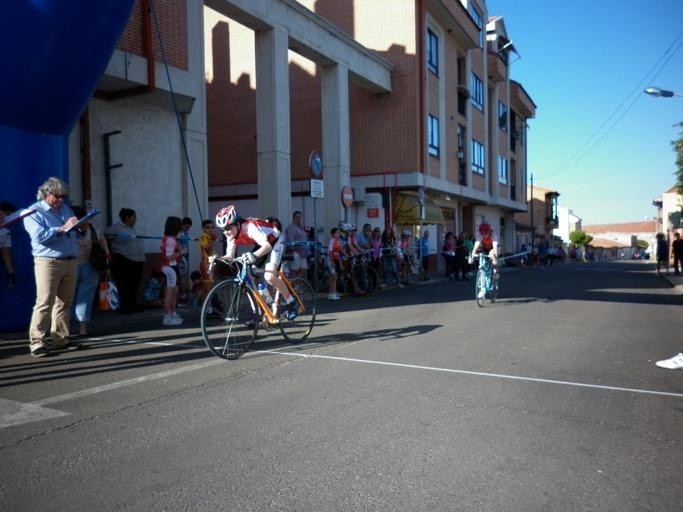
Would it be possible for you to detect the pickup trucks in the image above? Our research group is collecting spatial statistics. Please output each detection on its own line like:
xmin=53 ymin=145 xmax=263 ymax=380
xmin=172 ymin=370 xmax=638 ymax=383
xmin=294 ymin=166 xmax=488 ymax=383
xmin=68 ymin=205 xmax=167 ymax=309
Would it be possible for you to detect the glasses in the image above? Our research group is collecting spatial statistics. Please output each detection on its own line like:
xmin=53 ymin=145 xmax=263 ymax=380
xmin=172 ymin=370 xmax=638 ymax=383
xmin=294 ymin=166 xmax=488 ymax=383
xmin=220 ymin=227 xmax=230 ymax=232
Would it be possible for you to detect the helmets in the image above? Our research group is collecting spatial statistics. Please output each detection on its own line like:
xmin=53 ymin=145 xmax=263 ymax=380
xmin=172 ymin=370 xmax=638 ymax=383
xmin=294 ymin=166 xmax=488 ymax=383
xmin=215 ymin=204 xmax=239 ymax=228
xmin=340 ymin=223 xmax=353 ymax=232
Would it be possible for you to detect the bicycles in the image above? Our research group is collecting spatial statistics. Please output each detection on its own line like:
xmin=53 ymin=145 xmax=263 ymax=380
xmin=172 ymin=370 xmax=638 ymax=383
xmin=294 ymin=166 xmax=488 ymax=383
xmin=200 ymin=254 xmax=317 ymax=364
xmin=467 ymin=250 xmax=500 ymax=308
xmin=309 ymin=251 xmax=424 ymax=296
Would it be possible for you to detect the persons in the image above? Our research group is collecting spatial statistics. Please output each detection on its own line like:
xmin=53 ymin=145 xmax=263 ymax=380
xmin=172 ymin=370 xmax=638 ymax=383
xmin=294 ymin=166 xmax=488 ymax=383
xmin=22 ymin=176 xmax=89 ymax=358
xmin=672 ymin=232 xmax=683 ymax=276
xmin=655 ymin=233 xmax=670 ymax=277
xmin=0 ymin=200 xmax=18 ymax=290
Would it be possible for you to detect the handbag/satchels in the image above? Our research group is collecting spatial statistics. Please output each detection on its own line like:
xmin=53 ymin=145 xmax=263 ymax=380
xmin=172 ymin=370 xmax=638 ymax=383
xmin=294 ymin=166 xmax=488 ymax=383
xmin=89 ymin=242 xmax=108 ymax=273
xmin=99 ymin=281 xmax=121 ymax=310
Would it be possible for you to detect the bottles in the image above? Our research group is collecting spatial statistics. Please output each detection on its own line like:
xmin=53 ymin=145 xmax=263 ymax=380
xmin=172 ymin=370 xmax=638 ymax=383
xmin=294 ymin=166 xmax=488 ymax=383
xmin=256 ymin=282 xmax=273 ymax=306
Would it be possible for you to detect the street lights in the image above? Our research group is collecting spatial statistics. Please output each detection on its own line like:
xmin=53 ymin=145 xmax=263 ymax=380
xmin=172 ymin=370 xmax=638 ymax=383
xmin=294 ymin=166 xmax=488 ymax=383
xmin=645 ymin=87 xmax=682 ymax=102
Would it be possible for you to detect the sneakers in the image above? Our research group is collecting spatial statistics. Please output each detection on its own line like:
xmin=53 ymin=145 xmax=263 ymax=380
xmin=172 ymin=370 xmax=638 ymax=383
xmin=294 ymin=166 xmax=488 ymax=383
xmin=163 ymin=313 xmax=184 ymax=325
xmin=286 ymin=300 xmax=298 ymax=321
xmin=328 ymin=295 xmax=340 ymax=300
xmin=65 ymin=342 xmax=79 ymax=350
xmin=354 ymin=289 xmax=367 ymax=297
xmin=32 ymin=347 xmax=51 ymax=358
xmin=245 ymin=313 xmax=264 ymax=327
xmin=655 ymin=352 xmax=683 ymax=370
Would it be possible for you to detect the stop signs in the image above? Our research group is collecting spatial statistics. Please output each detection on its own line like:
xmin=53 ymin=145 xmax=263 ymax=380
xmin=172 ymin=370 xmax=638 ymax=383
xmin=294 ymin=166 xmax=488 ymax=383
xmin=342 ymin=185 xmax=353 ymax=208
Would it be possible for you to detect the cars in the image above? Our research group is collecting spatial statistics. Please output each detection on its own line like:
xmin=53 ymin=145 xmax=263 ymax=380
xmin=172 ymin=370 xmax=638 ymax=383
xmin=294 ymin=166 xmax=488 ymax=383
xmin=632 ymin=250 xmax=648 ymax=259
xmin=546 ymin=248 xmax=566 ymax=261
